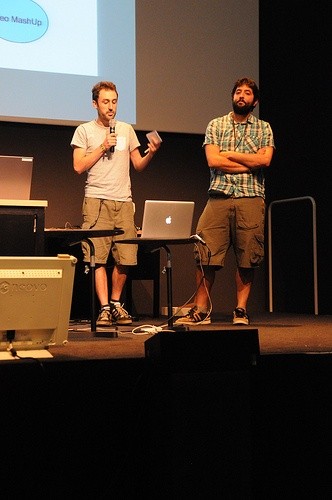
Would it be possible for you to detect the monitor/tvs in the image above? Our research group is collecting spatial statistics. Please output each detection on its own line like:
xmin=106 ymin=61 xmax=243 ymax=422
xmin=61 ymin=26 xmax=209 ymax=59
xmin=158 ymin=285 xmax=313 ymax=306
xmin=0 ymin=254 xmax=77 ymax=360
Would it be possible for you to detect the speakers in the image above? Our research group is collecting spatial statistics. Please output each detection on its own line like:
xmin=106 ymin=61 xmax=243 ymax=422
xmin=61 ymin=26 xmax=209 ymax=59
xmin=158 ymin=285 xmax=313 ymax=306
xmin=144 ymin=328 xmax=260 ymax=367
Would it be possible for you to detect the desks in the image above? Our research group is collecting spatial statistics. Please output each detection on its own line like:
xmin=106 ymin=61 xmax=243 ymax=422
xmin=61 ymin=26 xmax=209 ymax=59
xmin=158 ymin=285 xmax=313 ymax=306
xmin=45 ymin=229 xmax=126 ymax=338
xmin=0 ymin=198 xmax=48 ymax=257
xmin=112 ymin=236 xmax=199 ymax=330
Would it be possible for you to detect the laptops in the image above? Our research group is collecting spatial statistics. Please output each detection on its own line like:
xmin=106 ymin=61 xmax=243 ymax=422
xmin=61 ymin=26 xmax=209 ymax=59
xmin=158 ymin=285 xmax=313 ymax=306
xmin=140 ymin=200 xmax=194 ymax=239
xmin=0 ymin=156 xmax=33 ymax=200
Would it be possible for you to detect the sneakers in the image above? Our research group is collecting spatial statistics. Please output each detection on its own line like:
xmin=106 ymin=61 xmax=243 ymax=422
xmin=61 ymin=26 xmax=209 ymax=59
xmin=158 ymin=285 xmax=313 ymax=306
xmin=232 ymin=306 xmax=248 ymax=325
xmin=110 ymin=303 xmax=132 ymax=325
xmin=175 ymin=306 xmax=211 ymax=324
xmin=96 ymin=307 xmax=112 ymax=326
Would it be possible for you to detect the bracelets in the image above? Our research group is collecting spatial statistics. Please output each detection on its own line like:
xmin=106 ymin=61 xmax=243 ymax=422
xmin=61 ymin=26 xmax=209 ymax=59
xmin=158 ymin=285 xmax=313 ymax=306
xmin=100 ymin=144 xmax=106 ymax=153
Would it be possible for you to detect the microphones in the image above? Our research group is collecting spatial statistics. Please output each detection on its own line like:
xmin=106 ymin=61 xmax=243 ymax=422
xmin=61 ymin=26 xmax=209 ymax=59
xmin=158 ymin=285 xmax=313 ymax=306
xmin=109 ymin=119 xmax=117 ymax=152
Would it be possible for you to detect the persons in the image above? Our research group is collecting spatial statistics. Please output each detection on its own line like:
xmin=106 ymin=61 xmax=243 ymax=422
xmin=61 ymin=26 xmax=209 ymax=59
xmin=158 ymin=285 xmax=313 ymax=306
xmin=175 ymin=77 xmax=274 ymax=324
xmin=70 ymin=81 xmax=160 ymax=325
